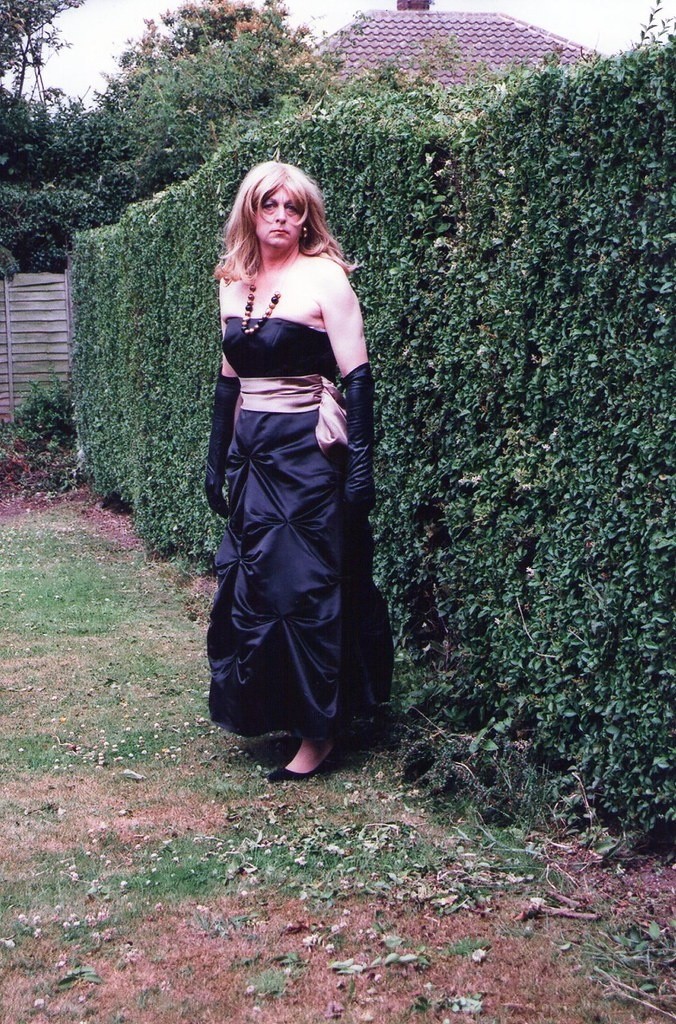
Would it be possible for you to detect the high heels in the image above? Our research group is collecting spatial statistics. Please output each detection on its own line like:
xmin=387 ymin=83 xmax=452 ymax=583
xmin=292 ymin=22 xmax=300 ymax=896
xmin=267 ymin=739 xmax=340 ymax=781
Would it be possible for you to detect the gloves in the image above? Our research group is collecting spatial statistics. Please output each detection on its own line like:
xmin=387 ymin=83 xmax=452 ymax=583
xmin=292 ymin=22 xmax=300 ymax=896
xmin=204 ymin=365 xmax=243 ymax=518
xmin=339 ymin=362 xmax=377 ymax=519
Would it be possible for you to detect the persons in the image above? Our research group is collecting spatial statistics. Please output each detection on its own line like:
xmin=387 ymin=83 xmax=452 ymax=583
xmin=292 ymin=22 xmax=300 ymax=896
xmin=207 ymin=162 xmax=394 ymax=781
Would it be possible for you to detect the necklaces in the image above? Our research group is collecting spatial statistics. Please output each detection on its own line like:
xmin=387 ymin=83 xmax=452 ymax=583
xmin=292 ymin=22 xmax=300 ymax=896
xmin=242 ymin=285 xmax=281 ymax=335
